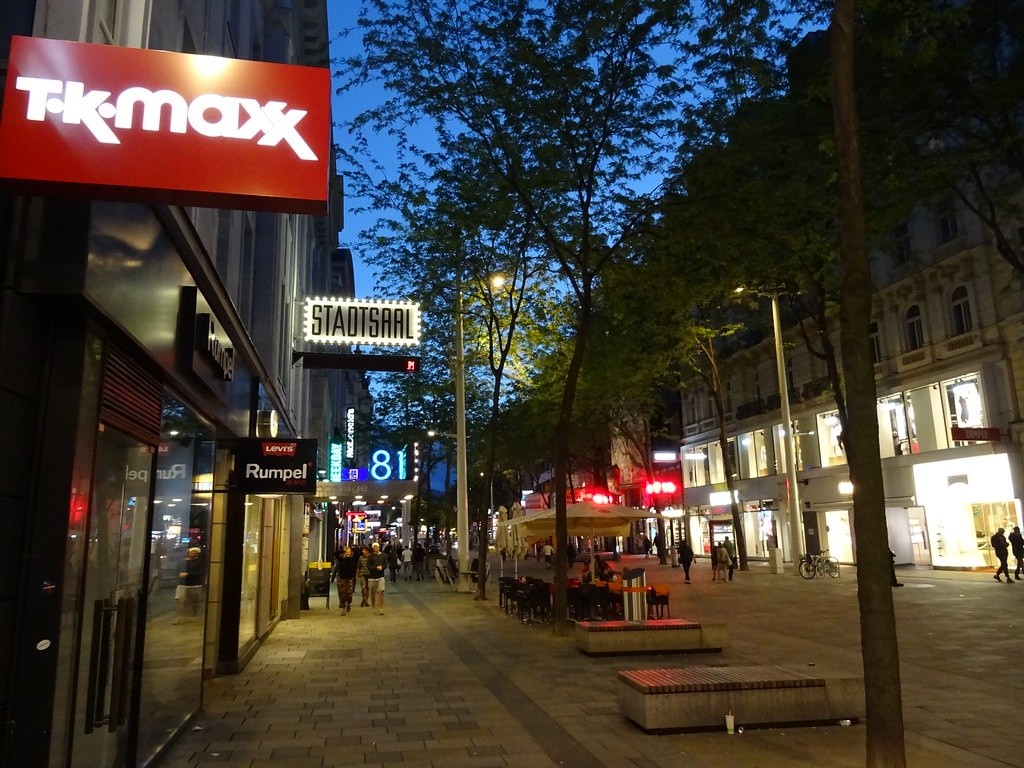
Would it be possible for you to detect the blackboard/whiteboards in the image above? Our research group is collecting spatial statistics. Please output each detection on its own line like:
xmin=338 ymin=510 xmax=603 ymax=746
xmin=307 ymin=567 xmax=330 ymax=596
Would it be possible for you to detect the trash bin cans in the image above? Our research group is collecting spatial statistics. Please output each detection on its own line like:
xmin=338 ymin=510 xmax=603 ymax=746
xmin=671 ymin=546 xmax=680 ymax=567
xmin=622 ymin=567 xmax=647 ymax=621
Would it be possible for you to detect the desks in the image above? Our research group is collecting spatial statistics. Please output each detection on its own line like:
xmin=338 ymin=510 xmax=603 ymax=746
xmin=460 ymin=572 xmax=476 ymax=592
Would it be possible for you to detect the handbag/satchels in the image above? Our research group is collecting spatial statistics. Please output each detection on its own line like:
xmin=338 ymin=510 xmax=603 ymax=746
xmin=726 ymin=553 xmax=732 ymax=567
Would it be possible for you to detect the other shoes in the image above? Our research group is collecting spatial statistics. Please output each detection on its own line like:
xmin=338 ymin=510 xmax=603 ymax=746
xmin=188 ymin=616 xmax=196 ymax=623
xmin=712 ymin=578 xmax=716 ymax=582
xmin=346 ymin=605 xmax=351 ymax=612
xmin=361 ymin=601 xmax=364 ymax=607
xmin=173 ymin=617 xmax=180 ymax=624
xmin=365 ymin=602 xmax=369 ymax=607
xmin=404 ymin=577 xmax=407 ymax=581
xmin=1007 ymin=578 xmax=1014 ymax=583
xmin=1015 ymin=577 xmax=1023 ymax=580
xmin=416 ymin=579 xmax=420 ymax=581
xmin=379 ymin=609 xmax=384 ymax=615
xmin=994 ymin=575 xmax=1002 ymax=582
xmin=341 ymin=611 xmax=346 ymax=616
xmin=421 ymin=574 xmax=424 ymax=580
xmin=685 ymin=581 xmax=691 ymax=584
xmin=409 ymin=575 xmax=412 ymax=580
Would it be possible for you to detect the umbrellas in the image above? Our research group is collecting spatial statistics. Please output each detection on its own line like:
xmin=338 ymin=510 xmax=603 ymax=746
xmin=500 ymin=503 xmax=666 ymax=583
xmin=510 ymin=501 xmax=529 ymax=580
xmin=495 ymin=506 xmax=510 ymax=578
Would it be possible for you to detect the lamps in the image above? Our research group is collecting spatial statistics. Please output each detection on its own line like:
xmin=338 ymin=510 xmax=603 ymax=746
xmin=317 ymin=467 xmax=327 ymax=474
xmin=316 ymin=476 xmax=330 ymax=483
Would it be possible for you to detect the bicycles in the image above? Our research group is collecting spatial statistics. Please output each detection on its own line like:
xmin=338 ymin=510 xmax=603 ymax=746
xmin=799 ymin=549 xmax=838 ymax=579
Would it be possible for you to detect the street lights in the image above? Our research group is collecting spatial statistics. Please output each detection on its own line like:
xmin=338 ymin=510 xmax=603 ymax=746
xmin=392 ymin=506 xmax=400 ymax=541
xmin=480 ymin=473 xmax=493 ymax=547
xmin=428 ymin=270 xmax=506 ymax=594
xmin=733 ymin=286 xmax=805 ymax=580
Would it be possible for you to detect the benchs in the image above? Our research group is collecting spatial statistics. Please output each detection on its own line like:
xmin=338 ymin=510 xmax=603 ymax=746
xmin=618 ymin=663 xmax=870 ymax=735
xmin=574 ymin=616 xmax=727 ymax=651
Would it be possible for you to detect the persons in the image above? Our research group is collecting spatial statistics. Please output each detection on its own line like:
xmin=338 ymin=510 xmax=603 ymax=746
xmin=644 ymin=533 xmax=663 ymax=559
xmin=991 ymin=528 xmax=1015 ymax=584
xmin=761 ymin=446 xmax=768 ymax=471
xmin=172 ymin=547 xmax=204 ymax=624
xmin=334 ymin=538 xmax=438 ymax=615
xmin=710 ymin=536 xmax=736 ymax=583
xmin=502 ymin=541 xmax=554 ymax=570
xmin=566 ymin=537 xmax=598 ymax=568
xmin=890 ymin=549 xmax=904 ymax=586
xmin=1008 ymin=527 xmax=1024 ymax=580
xmin=830 ymin=422 xmax=845 ymax=456
xmin=677 ymin=541 xmax=696 ymax=584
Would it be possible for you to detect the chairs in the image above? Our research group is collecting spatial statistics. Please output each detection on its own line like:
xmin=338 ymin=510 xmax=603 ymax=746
xmin=434 ymin=557 xmax=492 ymax=593
xmin=499 ymin=575 xmax=670 ymax=627
